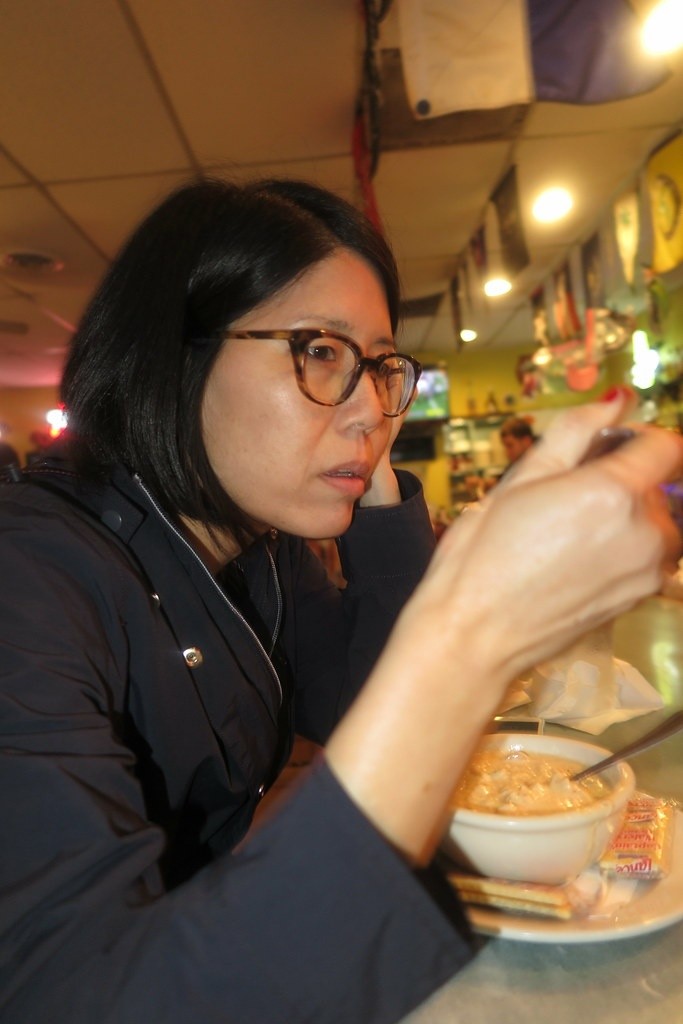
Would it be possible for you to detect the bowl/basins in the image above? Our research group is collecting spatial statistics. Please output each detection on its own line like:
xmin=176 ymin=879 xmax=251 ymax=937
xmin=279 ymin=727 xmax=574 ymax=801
xmin=441 ymin=732 xmax=635 ymax=887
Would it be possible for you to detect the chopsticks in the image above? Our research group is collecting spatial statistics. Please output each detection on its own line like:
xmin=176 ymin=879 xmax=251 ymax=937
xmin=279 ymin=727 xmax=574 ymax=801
xmin=448 ymin=876 xmax=571 ymax=920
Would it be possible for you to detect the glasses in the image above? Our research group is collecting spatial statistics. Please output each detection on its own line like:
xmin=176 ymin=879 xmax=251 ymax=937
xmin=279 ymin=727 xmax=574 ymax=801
xmin=227 ymin=325 xmax=420 ymax=418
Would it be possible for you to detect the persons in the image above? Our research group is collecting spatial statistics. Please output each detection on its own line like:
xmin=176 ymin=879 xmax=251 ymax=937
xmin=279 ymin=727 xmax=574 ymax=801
xmin=0 ymin=171 xmax=683 ymax=1023
xmin=465 ymin=417 xmax=542 ymax=500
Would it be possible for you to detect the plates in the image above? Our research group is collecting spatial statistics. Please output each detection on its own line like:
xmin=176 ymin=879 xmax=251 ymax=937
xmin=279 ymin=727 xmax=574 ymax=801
xmin=421 ymin=788 xmax=683 ymax=944
